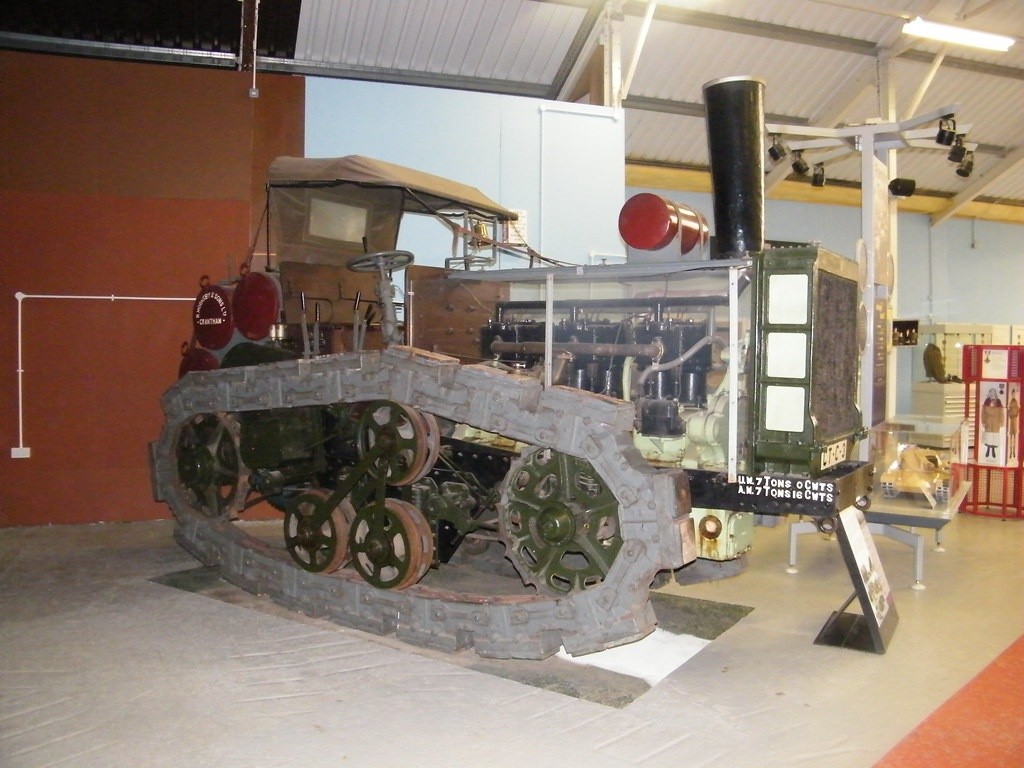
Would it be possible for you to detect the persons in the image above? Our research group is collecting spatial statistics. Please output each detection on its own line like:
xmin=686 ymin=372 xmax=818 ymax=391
xmin=1008 ymin=388 xmax=1018 ymax=458
xmin=982 ymin=389 xmax=1004 ymax=458
xmin=893 ymin=327 xmax=917 ymax=345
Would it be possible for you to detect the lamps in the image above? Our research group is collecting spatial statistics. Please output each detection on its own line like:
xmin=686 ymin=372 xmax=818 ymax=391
xmin=812 ymin=160 xmax=825 ymax=187
xmin=768 ymin=133 xmax=787 ymax=162
xmin=888 ymin=178 xmax=916 ymax=196
xmin=956 ymin=151 xmax=974 ymax=177
xmin=936 ymin=114 xmax=956 ymax=146
xmin=791 ymin=149 xmax=810 ymax=176
xmin=948 ymin=134 xmax=968 ymax=163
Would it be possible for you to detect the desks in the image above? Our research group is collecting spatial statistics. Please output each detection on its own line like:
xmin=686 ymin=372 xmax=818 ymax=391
xmin=786 ymin=480 xmax=973 ymax=590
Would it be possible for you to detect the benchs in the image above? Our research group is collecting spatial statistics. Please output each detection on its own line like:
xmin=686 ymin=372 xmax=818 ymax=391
xmin=280 ymin=263 xmax=405 ymax=354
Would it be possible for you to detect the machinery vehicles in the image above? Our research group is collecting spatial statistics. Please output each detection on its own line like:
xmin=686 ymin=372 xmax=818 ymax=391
xmin=147 ymin=76 xmax=875 ymax=661
